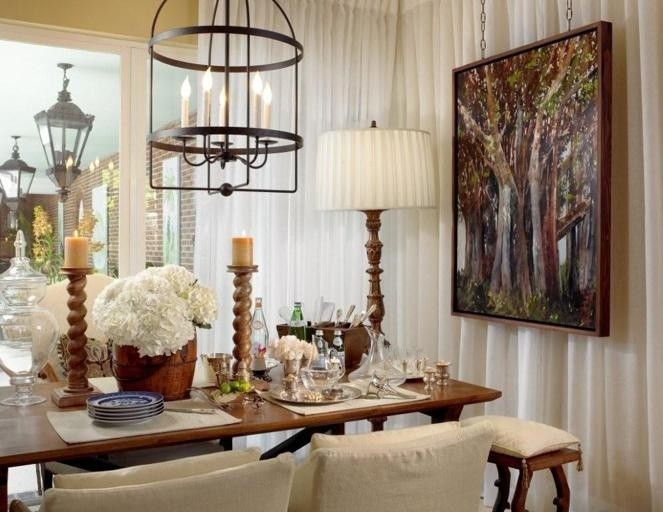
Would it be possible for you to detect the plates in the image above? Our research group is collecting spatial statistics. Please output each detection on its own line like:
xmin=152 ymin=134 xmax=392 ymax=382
xmin=87 ymin=391 xmax=165 ymax=426
xmin=268 ymin=383 xmax=362 ymax=404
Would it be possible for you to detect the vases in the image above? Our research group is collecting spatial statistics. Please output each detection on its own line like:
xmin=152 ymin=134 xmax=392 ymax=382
xmin=113 ymin=337 xmax=198 ymax=402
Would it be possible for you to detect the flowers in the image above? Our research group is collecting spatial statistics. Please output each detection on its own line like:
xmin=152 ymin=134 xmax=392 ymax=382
xmin=92 ymin=264 xmax=218 ymax=361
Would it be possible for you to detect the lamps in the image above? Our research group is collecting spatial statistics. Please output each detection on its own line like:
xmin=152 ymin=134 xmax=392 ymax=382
xmin=316 ymin=120 xmax=440 ymax=345
xmin=34 ymin=62 xmax=94 ymax=206
xmin=0 ymin=135 xmax=35 ymax=232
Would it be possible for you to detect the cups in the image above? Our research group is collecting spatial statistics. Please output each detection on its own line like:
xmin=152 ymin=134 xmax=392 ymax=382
xmin=200 ymin=353 xmax=232 ymax=384
xmin=300 ymin=370 xmax=340 ymax=396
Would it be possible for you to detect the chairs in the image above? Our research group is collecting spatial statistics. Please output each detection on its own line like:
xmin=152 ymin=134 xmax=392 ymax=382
xmin=9 ymin=452 xmax=294 ymax=512
xmin=291 ymin=416 xmax=495 ymax=512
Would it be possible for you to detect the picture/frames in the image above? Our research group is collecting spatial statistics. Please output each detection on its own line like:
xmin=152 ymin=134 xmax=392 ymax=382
xmin=161 ymin=156 xmax=182 ymax=269
xmin=450 ymin=22 xmax=611 ymax=338
xmin=88 ymin=185 xmax=108 ymax=273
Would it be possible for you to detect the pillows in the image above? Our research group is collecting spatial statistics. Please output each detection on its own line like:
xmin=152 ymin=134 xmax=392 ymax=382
xmin=459 ymin=414 xmax=585 ymax=487
xmin=52 ymin=447 xmax=261 ymax=490
xmin=312 ymin=420 xmax=458 ymax=457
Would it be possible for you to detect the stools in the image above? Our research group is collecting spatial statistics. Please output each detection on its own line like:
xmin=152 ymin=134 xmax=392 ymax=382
xmin=461 ymin=447 xmax=582 ymax=512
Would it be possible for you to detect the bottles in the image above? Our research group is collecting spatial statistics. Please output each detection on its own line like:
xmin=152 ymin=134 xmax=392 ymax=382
xmin=236 ymin=359 xmax=249 ymax=384
xmin=347 ymin=326 xmax=406 ymax=392
xmin=289 ymin=301 xmax=307 ymax=343
xmin=314 ymin=330 xmax=328 ymax=357
xmin=251 ymin=297 xmax=269 ymax=357
xmin=330 ymin=330 xmax=345 ymax=371
xmin=307 ymin=334 xmax=346 ymax=383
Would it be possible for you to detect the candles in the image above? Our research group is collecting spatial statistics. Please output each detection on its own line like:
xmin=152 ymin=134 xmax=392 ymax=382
xmin=219 ymin=89 xmax=227 ymax=124
xmin=233 ymin=228 xmax=254 ymax=266
xmin=180 ymin=75 xmax=191 ymax=129
xmin=252 ymin=70 xmax=263 ymax=129
xmin=63 ymin=230 xmax=90 ymax=271
xmin=262 ymin=80 xmax=274 ymax=128
xmin=200 ymin=67 xmax=213 ymax=126
